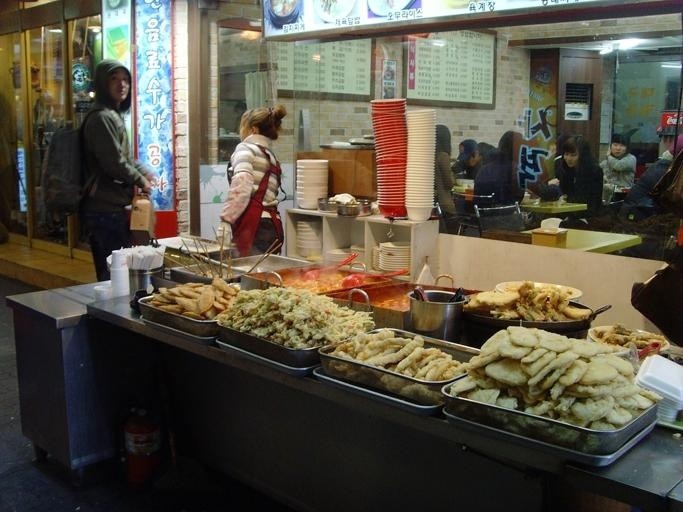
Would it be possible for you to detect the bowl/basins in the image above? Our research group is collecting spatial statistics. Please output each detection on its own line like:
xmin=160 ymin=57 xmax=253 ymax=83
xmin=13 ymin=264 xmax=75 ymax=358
xmin=337 ymin=201 xmax=359 ymax=220
xmin=295 ymin=220 xmax=323 ymax=258
xmin=404 ymin=110 xmax=437 ymax=221
xmin=296 ymin=159 xmax=329 ymax=212
xmin=355 ymin=199 xmax=372 ymax=216
xmin=370 ymin=98 xmax=407 ymax=219
xmin=318 ymin=197 xmax=335 ymax=211
xmin=406 ymin=290 xmax=469 ymax=344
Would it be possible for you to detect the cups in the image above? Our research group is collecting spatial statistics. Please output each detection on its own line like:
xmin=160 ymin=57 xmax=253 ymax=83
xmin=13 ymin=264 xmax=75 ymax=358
xmin=92 ymin=284 xmax=111 ymax=306
xmin=111 ymin=249 xmax=130 ymax=297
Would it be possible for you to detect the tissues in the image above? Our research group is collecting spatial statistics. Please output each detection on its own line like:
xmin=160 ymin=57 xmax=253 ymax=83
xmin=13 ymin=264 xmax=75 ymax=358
xmin=529 ymin=218 xmax=568 ymax=247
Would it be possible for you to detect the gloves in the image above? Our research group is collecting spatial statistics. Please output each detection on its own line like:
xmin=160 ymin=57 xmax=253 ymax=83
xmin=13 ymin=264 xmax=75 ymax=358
xmin=218 ymin=222 xmax=233 ymax=246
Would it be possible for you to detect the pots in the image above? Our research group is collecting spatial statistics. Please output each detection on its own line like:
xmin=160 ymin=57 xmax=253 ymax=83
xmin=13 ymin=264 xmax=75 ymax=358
xmin=267 ymin=0 xmax=300 ymax=29
xmin=463 ymin=299 xmax=612 ymax=333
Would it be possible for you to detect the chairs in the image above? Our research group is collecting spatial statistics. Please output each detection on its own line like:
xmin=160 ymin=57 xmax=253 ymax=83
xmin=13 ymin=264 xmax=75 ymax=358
xmin=473 ymin=201 xmax=523 ymax=238
xmin=451 ymin=189 xmax=495 ymax=236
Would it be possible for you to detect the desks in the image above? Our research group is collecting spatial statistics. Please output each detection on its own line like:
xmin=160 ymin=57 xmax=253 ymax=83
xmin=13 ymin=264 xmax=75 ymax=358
xmin=85 ymin=292 xmax=683 ymax=511
xmin=4 ymin=282 xmax=111 ymax=485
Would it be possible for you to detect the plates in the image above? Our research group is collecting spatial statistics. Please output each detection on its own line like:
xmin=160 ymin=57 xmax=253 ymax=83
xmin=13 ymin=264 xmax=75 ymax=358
xmin=312 ymin=1 xmax=359 ymax=24
xmin=493 ymin=279 xmax=584 ymax=307
xmin=351 ymin=242 xmax=365 ymax=267
xmin=442 ymin=405 xmax=661 ymax=468
xmin=588 ymin=325 xmax=670 ymax=356
xmin=139 ymin=315 xmax=220 ymax=346
xmin=318 ymin=143 xmax=375 ymax=151
xmin=215 ymin=336 xmax=319 ymax=379
xmin=348 ymin=138 xmax=376 ymax=145
xmin=313 ymin=367 xmax=443 ymax=417
xmin=366 ymin=0 xmax=420 ymax=18
xmin=326 ymin=248 xmax=351 ymax=265
xmin=380 ymin=242 xmax=411 ymax=272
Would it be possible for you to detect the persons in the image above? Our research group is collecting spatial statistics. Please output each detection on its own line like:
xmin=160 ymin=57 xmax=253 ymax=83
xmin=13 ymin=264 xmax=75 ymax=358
xmin=452 ymin=139 xmax=497 ymax=180
xmin=473 ymin=131 xmax=560 ymax=231
xmin=617 ymin=135 xmax=683 ymax=222
xmin=80 ymin=59 xmax=156 ymax=282
xmin=0 ymin=94 xmax=18 ymax=243
xmin=599 ymin=133 xmax=637 ymax=205
xmin=555 ymin=137 xmax=604 ymax=214
xmin=435 ymin=125 xmax=457 ymax=215
xmin=217 ymin=104 xmax=287 ymax=257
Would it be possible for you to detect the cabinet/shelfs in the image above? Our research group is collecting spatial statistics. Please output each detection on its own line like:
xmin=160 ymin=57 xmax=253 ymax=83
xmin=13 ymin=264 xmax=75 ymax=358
xmin=298 ymin=151 xmax=375 ymax=198
xmin=282 ymin=209 xmax=440 ymax=283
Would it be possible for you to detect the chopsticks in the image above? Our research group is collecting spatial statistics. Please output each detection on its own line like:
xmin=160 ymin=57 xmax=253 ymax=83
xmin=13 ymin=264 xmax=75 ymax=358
xmin=127 ymin=248 xmax=163 ymax=269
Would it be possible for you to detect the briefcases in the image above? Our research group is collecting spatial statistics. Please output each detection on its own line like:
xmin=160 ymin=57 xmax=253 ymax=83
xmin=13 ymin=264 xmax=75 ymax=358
xmin=130 ymin=191 xmax=154 ymax=242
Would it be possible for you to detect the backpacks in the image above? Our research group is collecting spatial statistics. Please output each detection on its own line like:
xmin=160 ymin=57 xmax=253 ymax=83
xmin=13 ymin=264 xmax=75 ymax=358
xmin=41 ymin=107 xmax=125 ymax=215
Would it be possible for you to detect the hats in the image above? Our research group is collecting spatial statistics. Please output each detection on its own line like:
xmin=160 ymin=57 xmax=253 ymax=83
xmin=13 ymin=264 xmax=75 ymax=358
xmin=457 ymin=139 xmax=479 ymax=161
